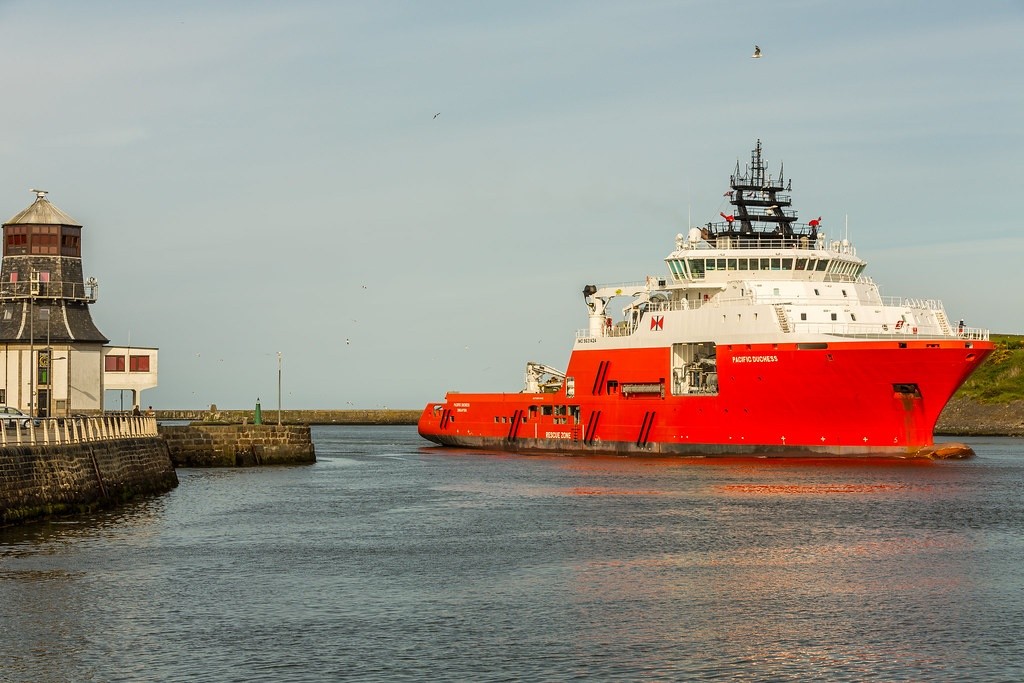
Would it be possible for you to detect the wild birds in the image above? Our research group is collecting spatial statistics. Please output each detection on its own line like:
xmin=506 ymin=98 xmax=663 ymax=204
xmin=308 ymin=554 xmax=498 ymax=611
xmin=751 ymin=45 xmax=763 ymax=58
xmin=346 ymin=285 xmax=367 ymax=345
xmin=433 ymin=113 xmax=440 ymax=119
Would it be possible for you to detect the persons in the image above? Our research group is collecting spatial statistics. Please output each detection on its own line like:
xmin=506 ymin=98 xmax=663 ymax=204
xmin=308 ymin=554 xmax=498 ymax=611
xmin=771 ymin=215 xmax=822 ymax=239
xmin=145 ymin=406 xmax=156 ymax=417
xmin=133 ymin=405 xmax=143 ymax=432
xmin=956 ymin=319 xmax=966 ymax=336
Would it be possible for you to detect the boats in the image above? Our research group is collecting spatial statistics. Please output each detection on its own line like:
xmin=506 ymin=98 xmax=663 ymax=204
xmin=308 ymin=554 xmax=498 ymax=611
xmin=415 ymin=139 xmax=998 ymax=465
